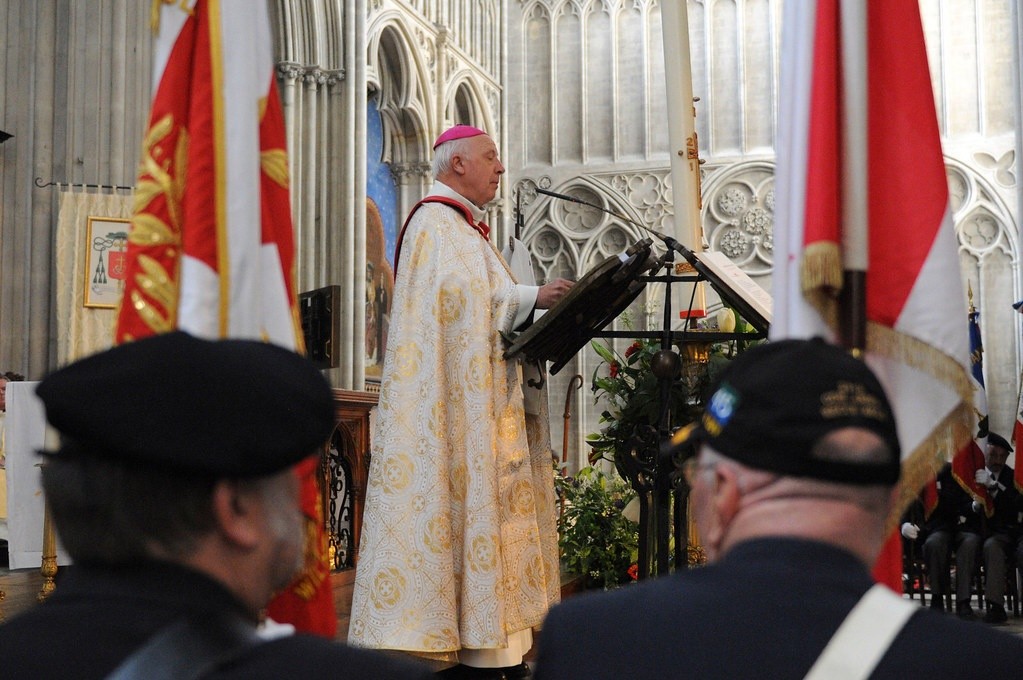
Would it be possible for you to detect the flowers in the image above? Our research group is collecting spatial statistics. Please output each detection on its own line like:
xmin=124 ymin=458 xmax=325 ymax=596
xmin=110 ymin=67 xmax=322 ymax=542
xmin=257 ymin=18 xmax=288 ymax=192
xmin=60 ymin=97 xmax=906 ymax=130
xmin=590 ymin=301 xmax=760 ymax=464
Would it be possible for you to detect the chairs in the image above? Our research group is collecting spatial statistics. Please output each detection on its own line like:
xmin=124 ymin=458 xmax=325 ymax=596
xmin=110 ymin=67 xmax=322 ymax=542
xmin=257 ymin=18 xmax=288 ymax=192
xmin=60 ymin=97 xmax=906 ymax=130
xmin=900 ymin=509 xmax=1023 ymax=617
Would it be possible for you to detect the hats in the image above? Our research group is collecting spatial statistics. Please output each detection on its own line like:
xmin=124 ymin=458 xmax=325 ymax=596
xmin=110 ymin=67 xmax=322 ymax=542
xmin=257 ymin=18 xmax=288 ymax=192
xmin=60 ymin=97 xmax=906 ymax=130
xmin=34 ymin=328 xmax=337 ymax=481
xmin=672 ymin=333 xmax=901 ymax=489
xmin=986 ymin=432 xmax=1013 ymax=453
xmin=433 ymin=123 xmax=486 ymax=150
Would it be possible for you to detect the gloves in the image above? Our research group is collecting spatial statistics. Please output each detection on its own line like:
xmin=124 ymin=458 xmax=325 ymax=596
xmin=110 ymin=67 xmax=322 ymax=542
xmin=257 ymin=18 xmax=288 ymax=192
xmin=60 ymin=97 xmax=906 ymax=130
xmin=975 ymin=470 xmax=995 ymax=489
xmin=970 ymin=494 xmax=984 ymax=512
xmin=902 ymin=523 xmax=921 ymax=539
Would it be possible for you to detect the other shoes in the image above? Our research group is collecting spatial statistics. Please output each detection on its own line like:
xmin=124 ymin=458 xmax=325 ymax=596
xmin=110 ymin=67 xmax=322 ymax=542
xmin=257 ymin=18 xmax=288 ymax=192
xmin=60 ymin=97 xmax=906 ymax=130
xmin=955 ymin=602 xmax=974 ymax=618
xmin=983 ymin=602 xmax=1006 ymax=621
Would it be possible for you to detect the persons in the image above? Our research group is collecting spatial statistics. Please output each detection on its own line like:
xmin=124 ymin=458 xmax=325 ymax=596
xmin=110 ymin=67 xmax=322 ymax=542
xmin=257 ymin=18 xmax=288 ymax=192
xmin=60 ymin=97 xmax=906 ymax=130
xmin=1 ymin=330 xmax=427 ymax=680
xmin=347 ymin=124 xmax=577 ymax=669
xmin=534 ymin=335 xmax=1023 ymax=679
xmin=0 ymin=376 xmax=11 ymax=521
xmin=902 ymin=440 xmax=1017 ymax=622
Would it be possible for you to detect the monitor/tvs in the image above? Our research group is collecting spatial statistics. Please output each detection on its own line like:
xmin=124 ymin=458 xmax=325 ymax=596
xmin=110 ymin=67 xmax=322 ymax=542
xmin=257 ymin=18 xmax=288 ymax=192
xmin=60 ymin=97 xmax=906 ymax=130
xmin=300 ymin=285 xmax=342 ymax=370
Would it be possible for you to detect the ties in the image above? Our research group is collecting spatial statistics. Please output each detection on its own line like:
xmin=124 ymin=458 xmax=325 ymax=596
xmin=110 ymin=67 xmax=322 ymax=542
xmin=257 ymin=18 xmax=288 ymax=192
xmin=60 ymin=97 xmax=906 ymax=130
xmin=990 ymin=475 xmax=996 ymax=493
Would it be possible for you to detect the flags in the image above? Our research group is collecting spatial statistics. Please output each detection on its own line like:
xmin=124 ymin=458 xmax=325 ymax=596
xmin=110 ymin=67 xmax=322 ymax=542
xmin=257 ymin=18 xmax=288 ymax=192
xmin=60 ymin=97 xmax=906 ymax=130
xmin=113 ymin=1 xmax=339 ymax=638
xmin=770 ymin=1 xmax=976 ymax=589
xmin=1012 ymin=301 xmax=1023 ymax=492
xmin=953 ymin=306 xmax=993 ymax=517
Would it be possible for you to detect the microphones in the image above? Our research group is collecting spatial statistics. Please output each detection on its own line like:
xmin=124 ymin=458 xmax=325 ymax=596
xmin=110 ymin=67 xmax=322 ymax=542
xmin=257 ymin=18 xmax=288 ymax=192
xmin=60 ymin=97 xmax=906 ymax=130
xmin=534 ymin=187 xmax=667 ymax=242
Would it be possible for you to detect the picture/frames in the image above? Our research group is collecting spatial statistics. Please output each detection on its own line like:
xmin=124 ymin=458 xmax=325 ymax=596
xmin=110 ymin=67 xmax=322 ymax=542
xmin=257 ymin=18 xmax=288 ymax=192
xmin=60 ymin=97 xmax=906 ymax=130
xmin=82 ymin=216 xmax=130 ymax=309
